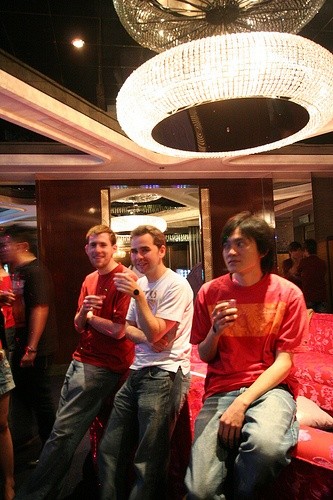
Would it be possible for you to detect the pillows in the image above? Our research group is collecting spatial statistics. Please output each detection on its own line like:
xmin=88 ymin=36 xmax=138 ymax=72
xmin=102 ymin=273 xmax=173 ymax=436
xmin=295 ymin=396 xmax=333 ymax=429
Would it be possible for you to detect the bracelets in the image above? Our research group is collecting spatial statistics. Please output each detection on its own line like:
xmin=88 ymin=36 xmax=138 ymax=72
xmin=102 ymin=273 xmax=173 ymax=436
xmin=25 ymin=346 xmax=38 ymax=354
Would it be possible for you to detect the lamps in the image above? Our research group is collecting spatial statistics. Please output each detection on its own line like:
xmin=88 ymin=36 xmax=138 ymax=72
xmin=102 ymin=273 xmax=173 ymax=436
xmin=116 ymin=32 xmax=333 ymax=159
xmin=110 ymin=193 xmax=166 ymax=237
xmin=112 ymin=0 xmax=326 ymax=52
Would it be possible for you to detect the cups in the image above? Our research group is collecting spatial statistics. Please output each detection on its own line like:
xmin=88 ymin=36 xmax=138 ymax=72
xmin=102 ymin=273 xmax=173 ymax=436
xmin=216 ymin=299 xmax=236 ymax=322
xmin=115 ymin=273 xmax=131 ymax=278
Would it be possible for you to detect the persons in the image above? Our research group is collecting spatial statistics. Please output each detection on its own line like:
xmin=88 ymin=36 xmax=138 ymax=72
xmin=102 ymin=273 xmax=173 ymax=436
xmin=281 ymin=242 xmax=304 ymax=290
xmin=12 ymin=225 xmax=139 ymax=500
xmin=183 ymin=210 xmax=307 ymax=500
xmin=98 ymin=227 xmax=194 ymax=500
xmin=0 ymin=223 xmax=52 ymax=500
xmin=292 ymin=238 xmax=328 ymax=313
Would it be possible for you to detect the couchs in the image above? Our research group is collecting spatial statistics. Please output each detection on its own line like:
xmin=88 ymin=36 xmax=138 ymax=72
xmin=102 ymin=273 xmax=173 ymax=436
xmin=186 ymin=314 xmax=333 ymax=500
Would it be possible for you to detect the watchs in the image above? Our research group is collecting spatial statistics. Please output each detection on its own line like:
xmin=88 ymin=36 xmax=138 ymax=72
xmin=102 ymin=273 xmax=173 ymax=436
xmin=134 ymin=289 xmax=141 ymax=299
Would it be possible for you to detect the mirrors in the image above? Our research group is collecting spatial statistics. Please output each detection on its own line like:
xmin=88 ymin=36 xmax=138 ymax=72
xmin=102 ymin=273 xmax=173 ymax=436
xmin=101 ymin=185 xmax=212 ymax=283
